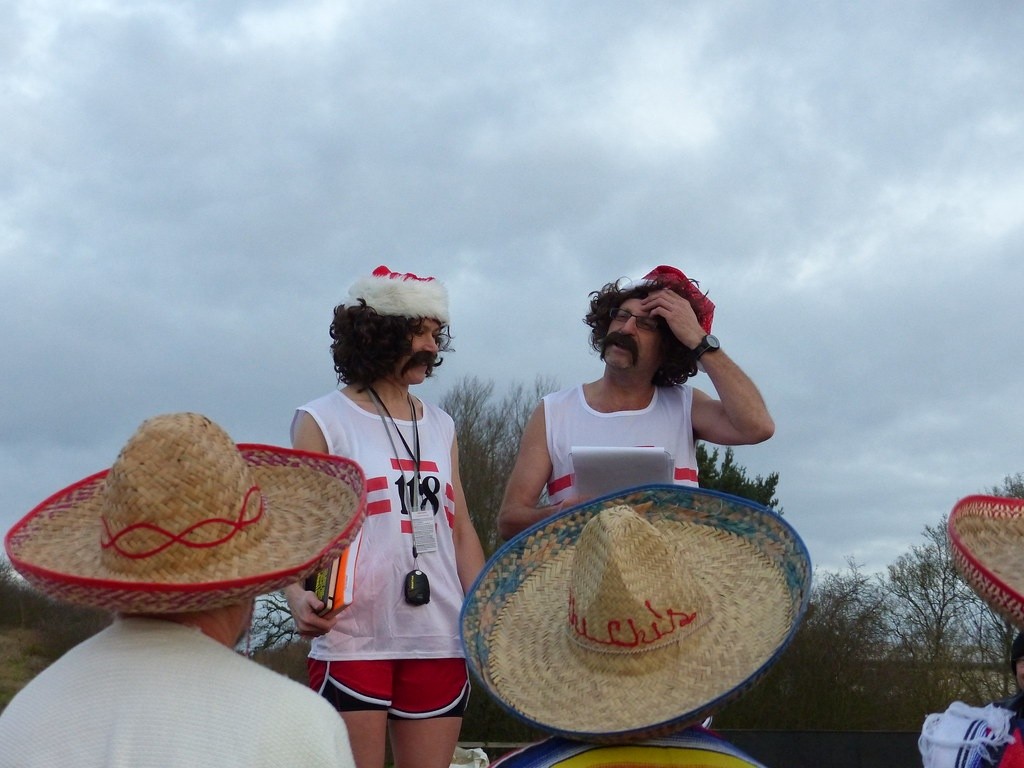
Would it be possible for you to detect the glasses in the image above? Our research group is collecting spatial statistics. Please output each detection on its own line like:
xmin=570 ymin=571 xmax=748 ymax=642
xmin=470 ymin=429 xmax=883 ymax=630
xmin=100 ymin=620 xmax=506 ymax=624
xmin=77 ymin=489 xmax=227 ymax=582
xmin=1012 ymin=659 xmax=1024 ymax=667
xmin=610 ymin=308 xmax=666 ymax=332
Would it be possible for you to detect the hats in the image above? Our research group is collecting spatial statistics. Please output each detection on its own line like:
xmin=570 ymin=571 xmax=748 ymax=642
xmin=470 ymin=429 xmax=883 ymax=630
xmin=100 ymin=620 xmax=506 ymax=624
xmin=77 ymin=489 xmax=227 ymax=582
xmin=347 ymin=266 xmax=449 ymax=326
xmin=1011 ymin=631 xmax=1024 ymax=670
xmin=5 ymin=412 xmax=367 ymax=612
xmin=621 ymin=265 xmax=715 ymax=337
xmin=458 ymin=484 xmax=812 ymax=743
xmin=949 ymin=496 xmax=1024 ymax=627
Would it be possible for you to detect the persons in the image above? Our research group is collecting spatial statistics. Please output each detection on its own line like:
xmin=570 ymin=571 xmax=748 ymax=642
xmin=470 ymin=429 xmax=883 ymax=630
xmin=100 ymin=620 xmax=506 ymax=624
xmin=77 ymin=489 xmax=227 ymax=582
xmin=458 ymin=483 xmax=807 ymax=768
xmin=497 ymin=265 xmax=776 ymax=542
xmin=284 ymin=267 xmax=487 ymax=768
xmin=917 ymin=494 xmax=1024 ymax=768
xmin=0 ymin=411 xmax=368 ymax=768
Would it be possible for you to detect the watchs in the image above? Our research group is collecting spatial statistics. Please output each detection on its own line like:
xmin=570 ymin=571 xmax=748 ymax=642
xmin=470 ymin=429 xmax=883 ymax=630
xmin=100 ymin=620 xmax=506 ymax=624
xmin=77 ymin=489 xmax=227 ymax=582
xmin=690 ymin=335 xmax=720 ymax=361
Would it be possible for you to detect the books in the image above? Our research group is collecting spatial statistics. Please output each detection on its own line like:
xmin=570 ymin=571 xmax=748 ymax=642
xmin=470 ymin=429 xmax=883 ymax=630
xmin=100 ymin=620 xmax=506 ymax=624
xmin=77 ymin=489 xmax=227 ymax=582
xmin=570 ymin=446 xmax=674 ymax=503
xmin=304 ymin=526 xmax=366 ymax=621
